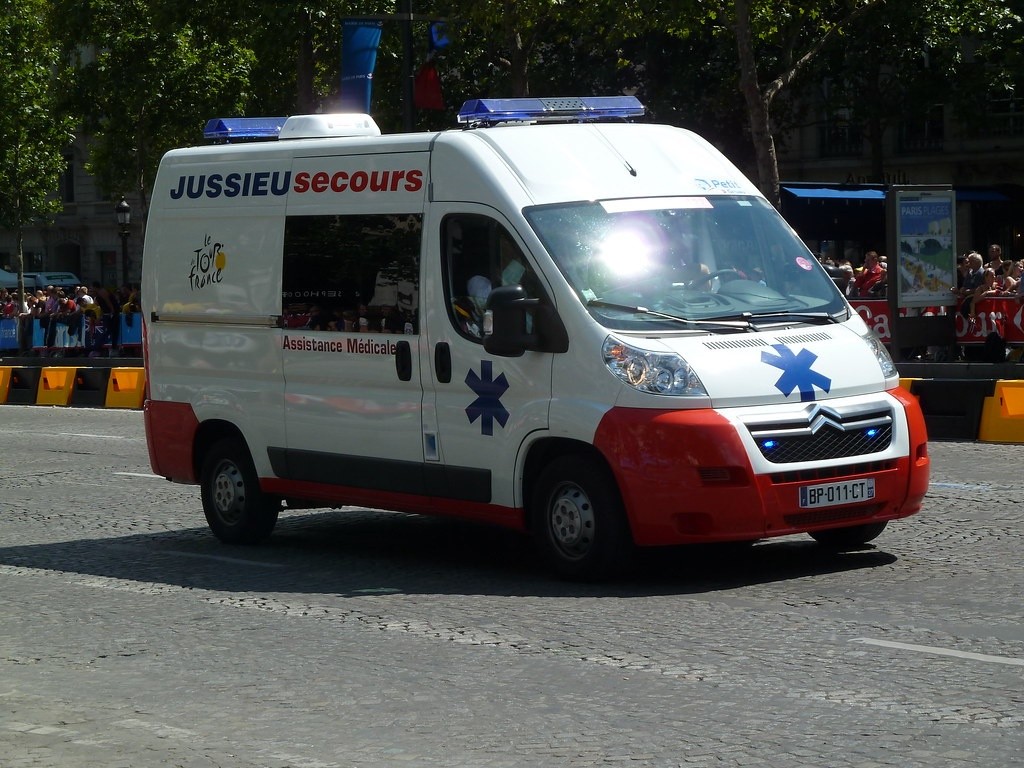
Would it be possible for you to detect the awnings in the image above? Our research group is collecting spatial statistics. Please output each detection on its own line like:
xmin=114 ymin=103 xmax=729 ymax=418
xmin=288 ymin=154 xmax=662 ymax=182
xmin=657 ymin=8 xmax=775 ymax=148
xmin=780 ymin=181 xmax=1024 ymax=204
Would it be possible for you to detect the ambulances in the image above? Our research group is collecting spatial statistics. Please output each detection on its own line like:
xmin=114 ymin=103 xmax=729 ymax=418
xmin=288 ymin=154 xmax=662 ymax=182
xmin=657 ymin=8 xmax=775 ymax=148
xmin=139 ymin=93 xmax=934 ymax=594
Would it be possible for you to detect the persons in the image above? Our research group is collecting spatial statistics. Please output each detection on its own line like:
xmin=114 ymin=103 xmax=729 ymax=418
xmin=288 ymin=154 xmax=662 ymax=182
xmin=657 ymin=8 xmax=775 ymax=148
xmin=950 ymin=243 xmax=1024 ymax=296
xmin=0 ymin=285 xmax=120 ymax=326
xmin=671 ymin=259 xmax=713 ymax=294
xmin=122 ymin=292 xmax=140 ymax=313
xmin=818 ymin=250 xmax=887 ymax=299
